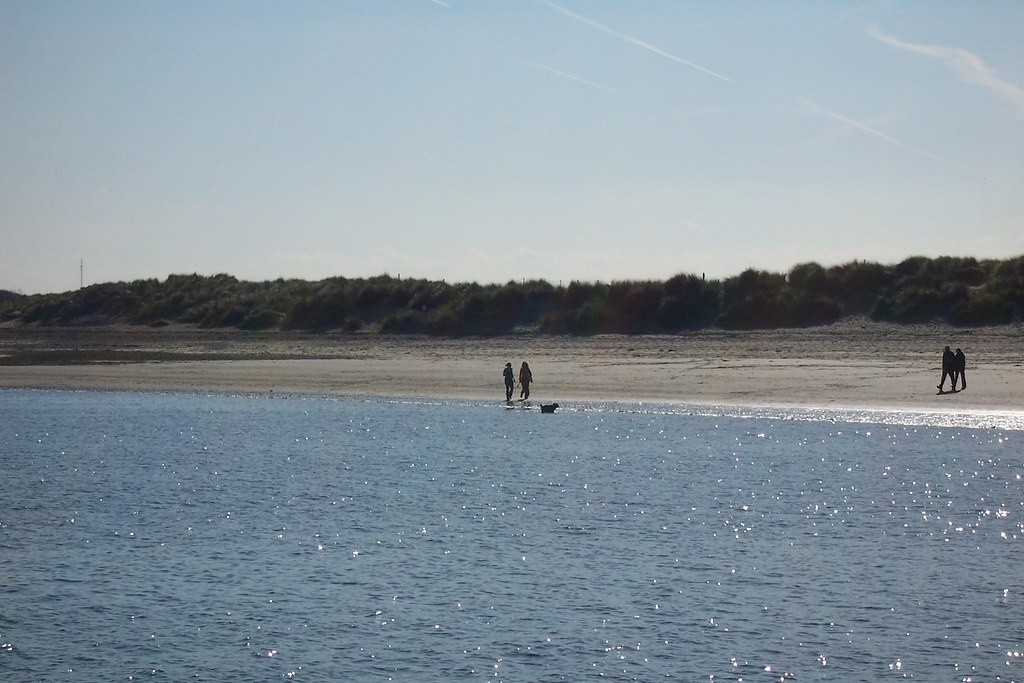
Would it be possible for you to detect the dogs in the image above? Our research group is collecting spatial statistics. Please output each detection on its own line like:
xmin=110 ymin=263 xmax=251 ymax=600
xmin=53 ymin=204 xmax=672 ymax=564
xmin=540 ymin=403 xmax=559 ymax=414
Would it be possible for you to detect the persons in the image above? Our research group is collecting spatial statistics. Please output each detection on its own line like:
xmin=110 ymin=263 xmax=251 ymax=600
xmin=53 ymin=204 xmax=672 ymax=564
xmin=519 ymin=362 xmax=533 ymax=399
xmin=951 ymin=348 xmax=966 ymax=389
xmin=936 ymin=346 xmax=954 ymax=390
xmin=503 ymin=363 xmax=515 ymax=400
